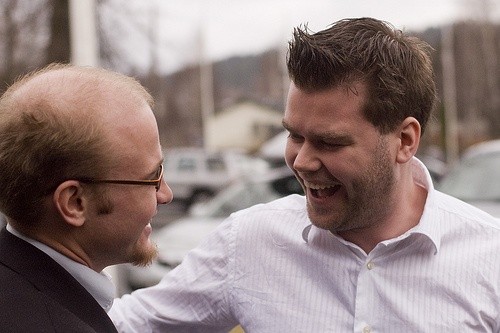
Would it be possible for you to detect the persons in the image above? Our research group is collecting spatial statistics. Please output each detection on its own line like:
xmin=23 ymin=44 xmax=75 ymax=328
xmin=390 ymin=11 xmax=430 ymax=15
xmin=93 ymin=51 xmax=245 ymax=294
xmin=0 ymin=59 xmax=176 ymax=333
xmin=104 ymin=14 xmax=500 ymax=333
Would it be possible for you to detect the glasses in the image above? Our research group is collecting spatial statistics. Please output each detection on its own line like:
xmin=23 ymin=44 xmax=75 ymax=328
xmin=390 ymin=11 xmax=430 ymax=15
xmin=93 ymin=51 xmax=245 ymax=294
xmin=78 ymin=164 xmax=165 ymax=192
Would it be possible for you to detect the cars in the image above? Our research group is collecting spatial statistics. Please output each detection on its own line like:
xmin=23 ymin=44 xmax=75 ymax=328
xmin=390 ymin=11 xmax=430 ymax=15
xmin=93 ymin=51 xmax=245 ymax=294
xmin=154 ymin=149 xmax=267 ymax=209
xmin=118 ymin=161 xmax=301 ymax=303
xmin=424 ymin=137 xmax=500 ymax=222
